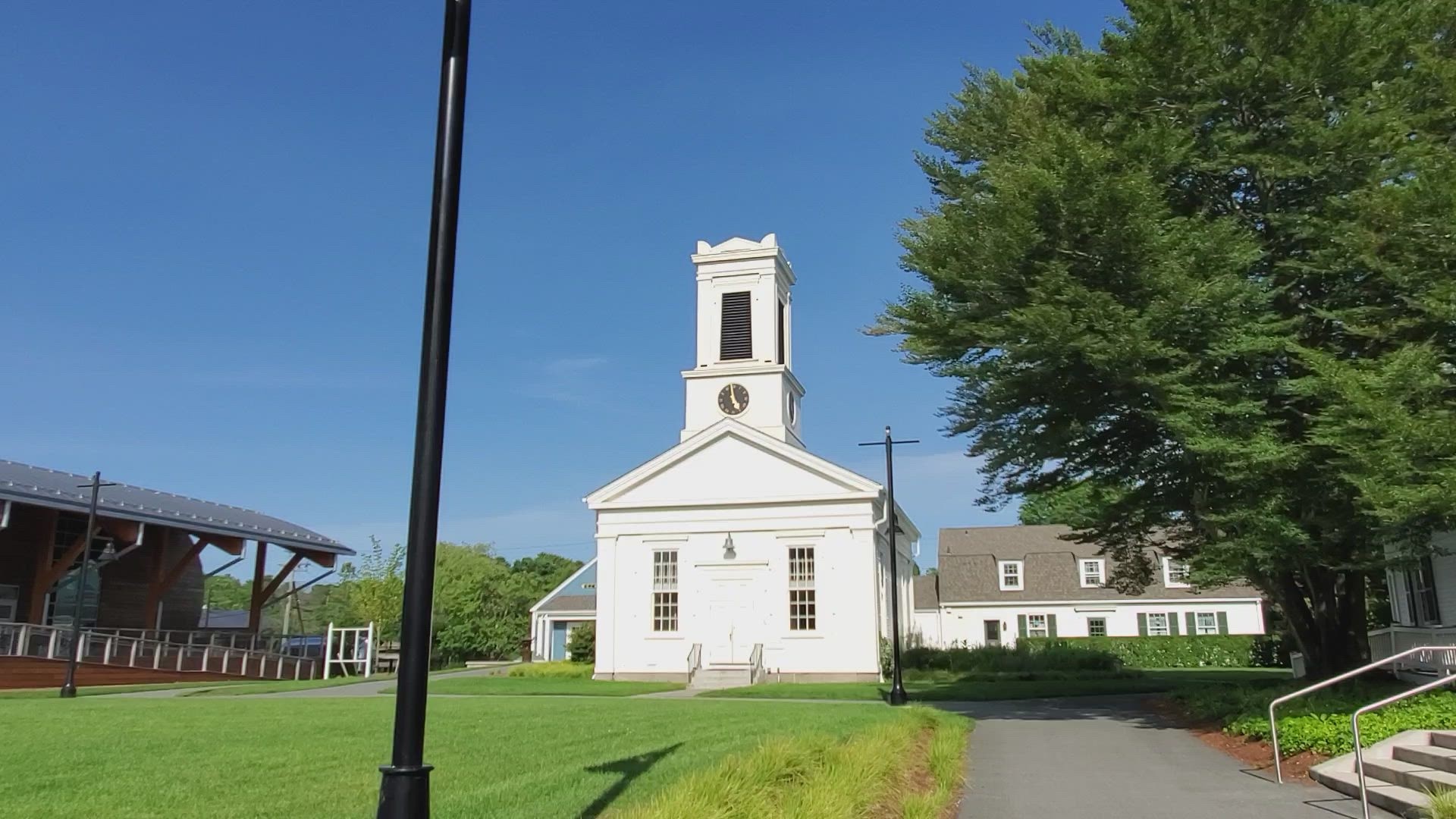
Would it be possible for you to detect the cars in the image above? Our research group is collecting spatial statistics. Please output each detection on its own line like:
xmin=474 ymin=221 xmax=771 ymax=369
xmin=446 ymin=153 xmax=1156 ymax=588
xmin=282 ymin=638 xmax=348 ymax=678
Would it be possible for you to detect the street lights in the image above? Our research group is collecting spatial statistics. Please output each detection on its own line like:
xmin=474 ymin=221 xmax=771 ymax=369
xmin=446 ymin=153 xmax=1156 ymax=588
xmin=857 ymin=427 xmax=920 ymax=705
xmin=59 ymin=471 xmax=115 ymax=698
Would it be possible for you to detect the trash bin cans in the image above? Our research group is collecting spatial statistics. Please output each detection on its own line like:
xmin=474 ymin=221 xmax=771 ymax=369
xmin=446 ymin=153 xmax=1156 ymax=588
xmin=522 ymin=639 xmax=531 ymax=662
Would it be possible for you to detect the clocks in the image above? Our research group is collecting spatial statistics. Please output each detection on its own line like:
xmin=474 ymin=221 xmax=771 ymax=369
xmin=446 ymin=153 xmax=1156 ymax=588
xmin=716 ymin=380 xmax=751 ymax=417
